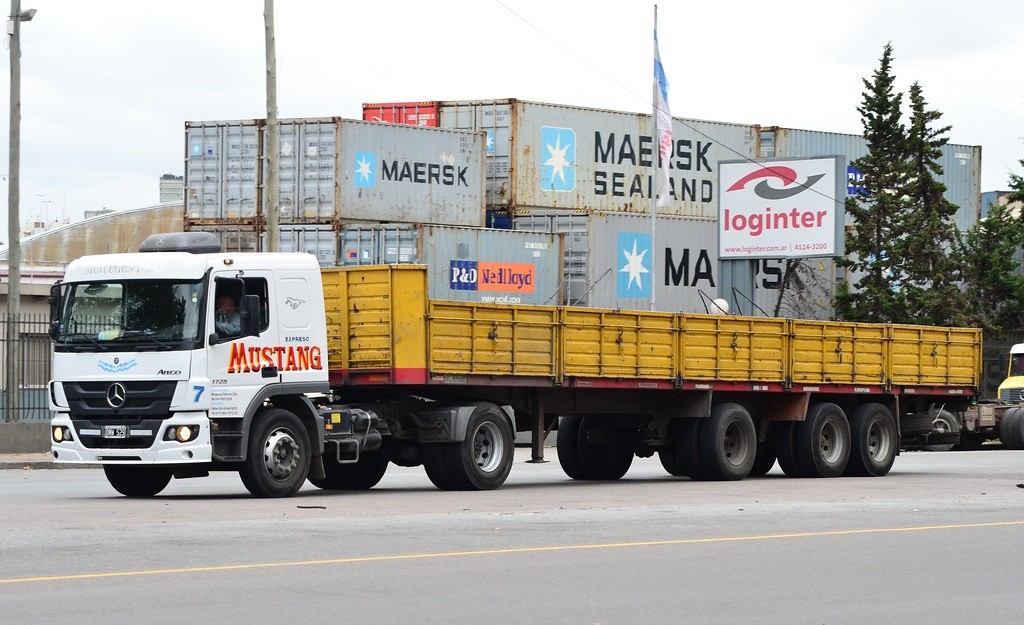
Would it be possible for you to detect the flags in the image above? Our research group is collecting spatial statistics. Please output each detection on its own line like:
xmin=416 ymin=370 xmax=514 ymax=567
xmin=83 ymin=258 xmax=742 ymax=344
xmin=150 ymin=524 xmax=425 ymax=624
xmin=653 ymin=31 xmax=673 ymax=208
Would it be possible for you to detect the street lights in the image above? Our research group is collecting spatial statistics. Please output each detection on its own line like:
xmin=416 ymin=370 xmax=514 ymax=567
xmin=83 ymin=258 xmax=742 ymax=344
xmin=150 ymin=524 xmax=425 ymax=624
xmin=7 ymin=0 xmax=37 ymax=422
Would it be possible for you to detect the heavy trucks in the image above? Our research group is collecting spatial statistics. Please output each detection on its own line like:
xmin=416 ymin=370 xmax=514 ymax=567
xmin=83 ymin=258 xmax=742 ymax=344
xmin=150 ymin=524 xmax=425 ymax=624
xmin=901 ymin=345 xmax=1024 ymax=451
xmin=44 ymin=233 xmax=984 ymax=498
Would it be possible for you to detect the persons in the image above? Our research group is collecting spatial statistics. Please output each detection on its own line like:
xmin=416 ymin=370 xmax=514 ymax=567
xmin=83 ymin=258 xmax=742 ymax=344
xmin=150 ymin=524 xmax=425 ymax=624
xmin=214 ymin=292 xmax=242 ymax=338
